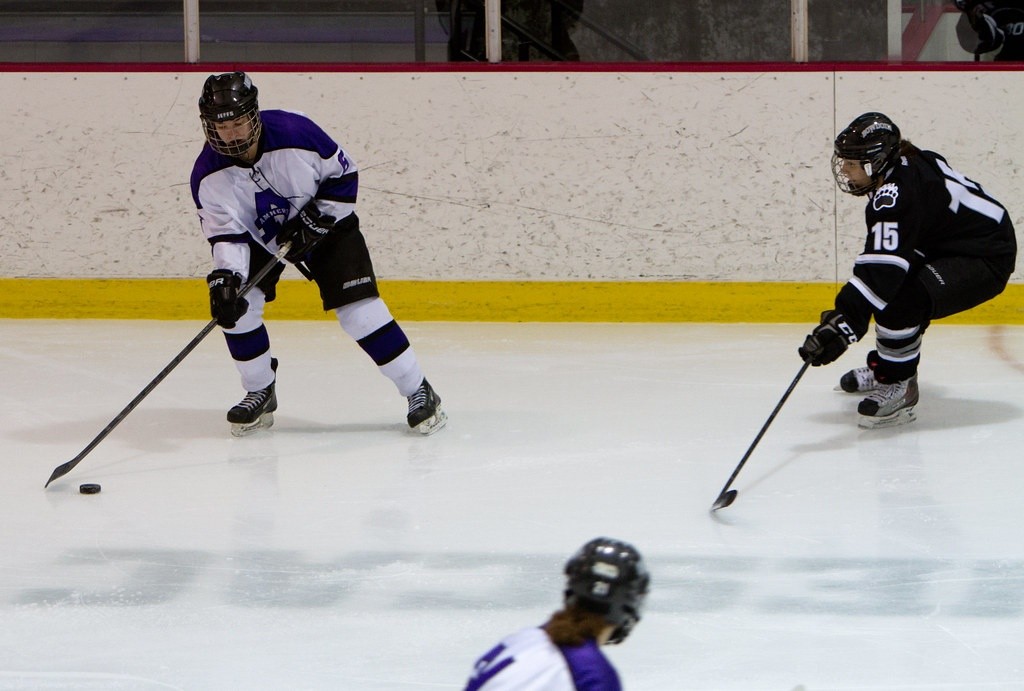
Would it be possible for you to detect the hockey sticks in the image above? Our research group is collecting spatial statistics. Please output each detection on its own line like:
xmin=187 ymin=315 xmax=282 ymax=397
xmin=43 ymin=233 xmax=297 ymax=488
xmin=709 ymin=355 xmax=815 ymax=515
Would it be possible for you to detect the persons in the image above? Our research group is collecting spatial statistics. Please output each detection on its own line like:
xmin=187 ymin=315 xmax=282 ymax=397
xmin=797 ymin=110 xmax=1018 ymax=431
xmin=446 ymin=2 xmax=583 ymax=62
xmin=189 ymin=70 xmax=450 ymax=436
xmin=953 ymin=1 xmax=1024 ymax=63
xmin=465 ymin=535 xmax=654 ymax=691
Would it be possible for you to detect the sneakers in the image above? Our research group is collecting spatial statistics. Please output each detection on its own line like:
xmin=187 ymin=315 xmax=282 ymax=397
xmin=840 ymin=350 xmax=889 ymax=393
xmin=857 ymin=370 xmax=919 ymax=429
xmin=227 ymin=357 xmax=278 ymax=437
xmin=406 ymin=377 xmax=448 ymax=436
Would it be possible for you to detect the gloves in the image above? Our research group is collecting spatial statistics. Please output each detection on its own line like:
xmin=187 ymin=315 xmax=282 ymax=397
xmin=206 ymin=268 xmax=249 ymax=329
xmin=275 ymin=198 xmax=337 ymax=264
xmin=797 ymin=308 xmax=858 ymax=366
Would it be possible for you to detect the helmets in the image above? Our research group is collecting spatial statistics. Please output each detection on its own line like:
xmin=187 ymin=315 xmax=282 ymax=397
xmin=834 ymin=112 xmax=901 ymax=198
xmin=563 ymin=538 xmax=649 ymax=644
xmin=198 ymin=70 xmax=259 ymax=155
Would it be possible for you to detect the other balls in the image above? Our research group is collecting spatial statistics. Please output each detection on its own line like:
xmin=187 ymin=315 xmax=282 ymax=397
xmin=80 ymin=483 xmax=101 ymax=494
